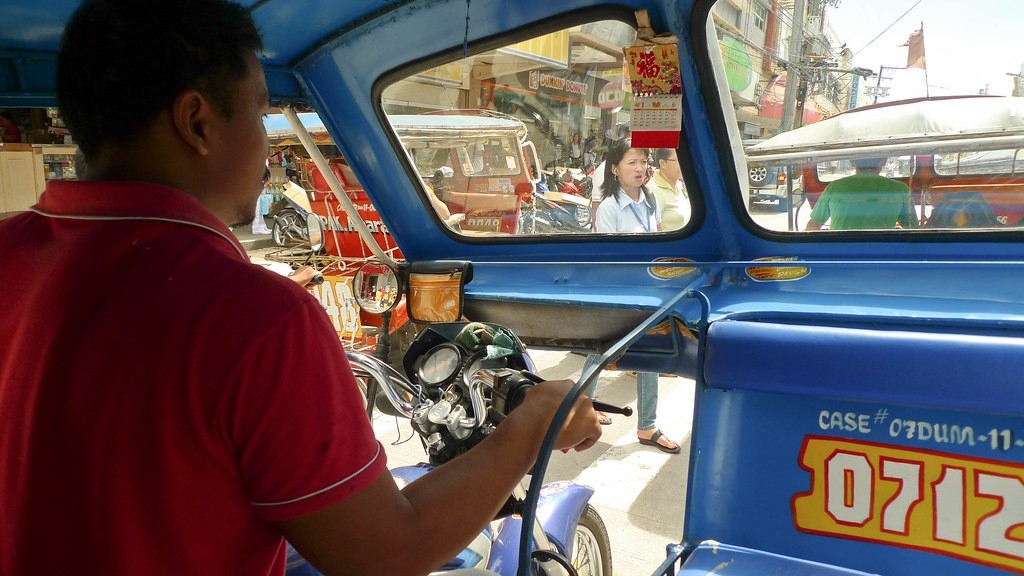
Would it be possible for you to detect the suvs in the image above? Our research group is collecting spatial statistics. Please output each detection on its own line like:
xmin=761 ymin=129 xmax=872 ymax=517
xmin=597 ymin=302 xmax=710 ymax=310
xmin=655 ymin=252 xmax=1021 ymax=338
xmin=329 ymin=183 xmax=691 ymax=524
xmin=741 ymin=139 xmax=805 ymax=212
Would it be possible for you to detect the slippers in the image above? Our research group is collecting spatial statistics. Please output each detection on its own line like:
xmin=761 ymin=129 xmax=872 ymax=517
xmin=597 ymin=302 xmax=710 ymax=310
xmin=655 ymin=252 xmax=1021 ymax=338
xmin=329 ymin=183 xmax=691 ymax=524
xmin=639 ymin=430 xmax=681 ymax=452
xmin=599 ymin=412 xmax=612 ymax=424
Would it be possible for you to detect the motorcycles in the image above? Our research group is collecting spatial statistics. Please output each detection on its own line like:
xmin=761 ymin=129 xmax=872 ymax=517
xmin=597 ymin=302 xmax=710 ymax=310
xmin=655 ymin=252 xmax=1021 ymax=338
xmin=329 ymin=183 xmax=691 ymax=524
xmin=524 ymin=159 xmax=596 ymax=234
xmin=746 ymin=94 xmax=1024 ymax=232
xmin=265 ymin=108 xmax=542 ymax=349
xmin=260 ymin=204 xmax=309 ymax=247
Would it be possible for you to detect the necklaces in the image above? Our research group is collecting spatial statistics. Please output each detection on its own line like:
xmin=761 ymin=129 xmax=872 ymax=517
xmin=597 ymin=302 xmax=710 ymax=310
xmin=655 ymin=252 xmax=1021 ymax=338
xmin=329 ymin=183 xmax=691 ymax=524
xmin=629 ymin=203 xmax=650 ymax=232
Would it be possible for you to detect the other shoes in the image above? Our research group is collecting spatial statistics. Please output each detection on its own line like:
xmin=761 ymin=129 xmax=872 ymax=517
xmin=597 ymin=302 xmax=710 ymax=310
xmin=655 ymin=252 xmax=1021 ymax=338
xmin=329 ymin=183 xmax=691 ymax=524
xmin=252 ymin=226 xmax=273 ymax=234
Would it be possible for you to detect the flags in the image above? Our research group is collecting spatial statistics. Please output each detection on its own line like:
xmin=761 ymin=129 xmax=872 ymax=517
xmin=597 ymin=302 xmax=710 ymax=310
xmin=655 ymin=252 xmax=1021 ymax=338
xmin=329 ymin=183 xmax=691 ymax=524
xmin=907 ymin=32 xmax=924 ymax=68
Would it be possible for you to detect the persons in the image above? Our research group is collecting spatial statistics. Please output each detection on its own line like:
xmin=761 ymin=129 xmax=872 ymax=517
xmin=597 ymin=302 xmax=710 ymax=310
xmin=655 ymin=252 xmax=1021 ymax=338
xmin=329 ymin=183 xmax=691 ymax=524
xmin=370 ymin=283 xmax=377 ymax=301
xmin=625 ymin=148 xmax=692 ymax=377
xmin=805 ymin=158 xmax=919 ymax=229
xmin=0 ymin=1 xmax=602 ymax=576
xmin=581 ymin=138 xmax=680 ymax=453
xmin=288 ymin=265 xmax=323 ymax=291
xmin=584 ymin=129 xmax=598 ymax=166
xmin=569 ymin=133 xmax=583 ymax=167
xmin=601 ymin=130 xmax=617 ymax=162
